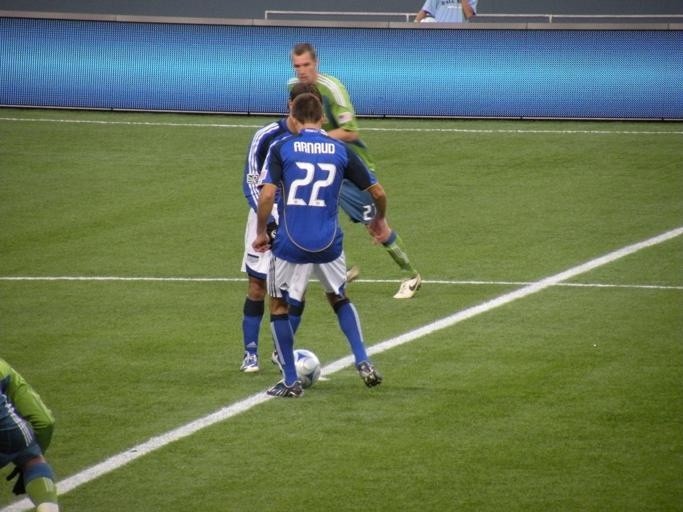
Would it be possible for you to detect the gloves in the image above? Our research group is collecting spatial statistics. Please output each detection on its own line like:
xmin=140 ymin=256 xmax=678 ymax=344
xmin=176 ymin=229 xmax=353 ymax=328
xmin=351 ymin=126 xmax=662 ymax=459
xmin=6 ymin=465 xmax=26 ymax=496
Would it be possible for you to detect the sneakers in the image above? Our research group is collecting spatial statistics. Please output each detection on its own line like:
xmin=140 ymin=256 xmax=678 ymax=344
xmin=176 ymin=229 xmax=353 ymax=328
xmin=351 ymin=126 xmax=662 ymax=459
xmin=240 ymin=351 xmax=260 ymax=373
xmin=393 ymin=273 xmax=424 ymax=299
xmin=345 ymin=265 xmax=360 ymax=284
xmin=266 ymin=379 xmax=305 ymax=399
xmin=357 ymin=362 xmax=383 ymax=388
xmin=271 ymin=350 xmax=287 ymax=376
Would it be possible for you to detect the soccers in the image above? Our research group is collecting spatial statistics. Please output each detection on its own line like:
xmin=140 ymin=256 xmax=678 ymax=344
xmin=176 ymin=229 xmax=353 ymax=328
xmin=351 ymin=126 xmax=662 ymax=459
xmin=293 ymin=349 xmax=320 ymax=389
xmin=420 ymin=17 xmax=436 ymax=22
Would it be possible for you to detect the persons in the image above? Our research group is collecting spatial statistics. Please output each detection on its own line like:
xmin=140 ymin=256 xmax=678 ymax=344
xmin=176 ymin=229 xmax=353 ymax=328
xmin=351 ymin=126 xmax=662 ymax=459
xmin=414 ymin=0 xmax=478 ymax=23
xmin=0 ymin=357 xmax=59 ymax=512
xmin=286 ymin=43 xmax=421 ymax=298
xmin=251 ymin=93 xmax=389 ymax=397
xmin=241 ymin=81 xmax=323 ymax=373
